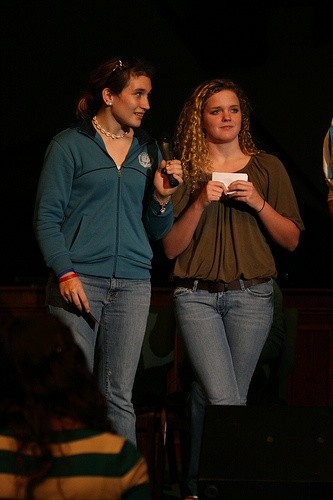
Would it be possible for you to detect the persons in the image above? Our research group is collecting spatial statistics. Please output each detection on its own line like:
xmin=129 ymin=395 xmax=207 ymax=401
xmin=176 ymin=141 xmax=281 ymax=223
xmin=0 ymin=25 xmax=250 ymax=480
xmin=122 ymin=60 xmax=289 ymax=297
xmin=164 ymin=78 xmax=306 ymax=500
xmin=35 ymin=56 xmax=184 ymax=444
xmin=322 ymin=118 xmax=333 ymax=216
xmin=0 ymin=306 xmax=156 ymax=500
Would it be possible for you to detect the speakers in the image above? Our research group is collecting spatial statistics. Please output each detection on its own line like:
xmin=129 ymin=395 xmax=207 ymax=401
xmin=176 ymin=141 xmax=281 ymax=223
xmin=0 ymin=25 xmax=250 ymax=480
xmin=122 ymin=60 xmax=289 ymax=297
xmin=195 ymin=403 xmax=333 ymax=500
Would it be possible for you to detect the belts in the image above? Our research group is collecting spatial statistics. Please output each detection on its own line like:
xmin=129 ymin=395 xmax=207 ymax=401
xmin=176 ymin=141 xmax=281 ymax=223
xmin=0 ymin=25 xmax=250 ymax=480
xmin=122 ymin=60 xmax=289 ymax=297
xmin=178 ymin=278 xmax=270 ymax=293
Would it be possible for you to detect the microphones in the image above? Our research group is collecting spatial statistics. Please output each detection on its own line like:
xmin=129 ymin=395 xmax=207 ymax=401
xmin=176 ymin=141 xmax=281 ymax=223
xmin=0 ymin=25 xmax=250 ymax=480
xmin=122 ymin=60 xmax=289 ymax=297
xmin=158 ymin=132 xmax=178 ymax=187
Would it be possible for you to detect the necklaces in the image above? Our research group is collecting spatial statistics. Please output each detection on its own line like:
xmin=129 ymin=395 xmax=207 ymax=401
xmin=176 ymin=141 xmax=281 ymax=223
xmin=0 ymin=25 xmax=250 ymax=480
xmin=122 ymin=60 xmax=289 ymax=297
xmin=92 ymin=115 xmax=131 ymax=139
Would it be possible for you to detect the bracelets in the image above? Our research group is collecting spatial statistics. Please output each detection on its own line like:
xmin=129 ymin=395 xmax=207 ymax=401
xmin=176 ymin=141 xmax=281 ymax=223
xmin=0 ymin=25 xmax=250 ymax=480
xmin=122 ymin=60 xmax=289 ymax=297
xmin=57 ymin=268 xmax=78 ymax=283
xmin=256 ymin=200 xmax=266 ymax=213
